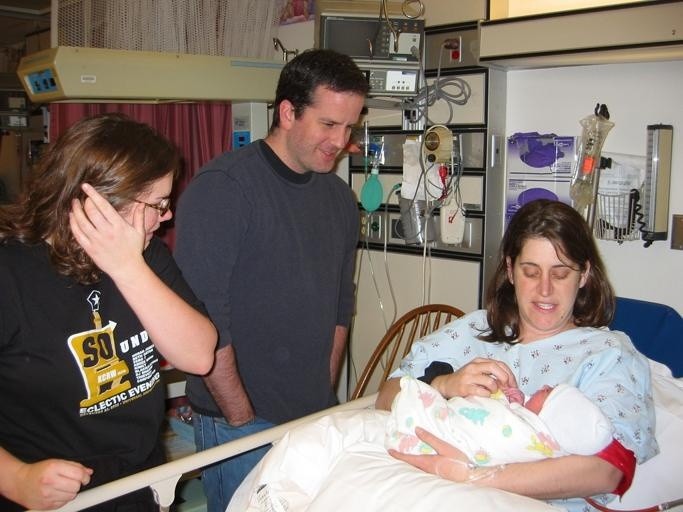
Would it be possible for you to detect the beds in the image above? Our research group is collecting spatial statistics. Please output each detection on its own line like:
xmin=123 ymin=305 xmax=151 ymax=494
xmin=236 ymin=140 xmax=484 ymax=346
xmin=28 ymin=296 xmax=683 ymax=512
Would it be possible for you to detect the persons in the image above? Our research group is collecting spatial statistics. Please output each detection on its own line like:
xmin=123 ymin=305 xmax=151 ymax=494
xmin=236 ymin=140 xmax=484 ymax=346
xmin=1 ymin=112 xmax=221 ymax=510
xmin=503 ymin=381 xmax=617 ymax=455
xmin=171 ymin=47 xmax=371 ymax=512
xmin=374 ymin=200 xmax=660 ymax=502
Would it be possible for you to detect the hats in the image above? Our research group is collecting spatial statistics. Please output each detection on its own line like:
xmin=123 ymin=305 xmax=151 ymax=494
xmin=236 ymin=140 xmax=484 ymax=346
xmin=537 ymin=384 xmax=617 ymax=455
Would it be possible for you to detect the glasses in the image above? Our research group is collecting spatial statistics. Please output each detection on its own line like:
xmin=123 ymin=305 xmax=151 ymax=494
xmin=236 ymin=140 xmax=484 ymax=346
xmin=129 ymin=199 xmax=170 ymax=217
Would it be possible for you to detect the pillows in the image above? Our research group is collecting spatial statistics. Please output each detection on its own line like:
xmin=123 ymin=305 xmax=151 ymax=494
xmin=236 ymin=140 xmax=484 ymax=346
xmin=591 ymin=297 xmax=683 ymax=511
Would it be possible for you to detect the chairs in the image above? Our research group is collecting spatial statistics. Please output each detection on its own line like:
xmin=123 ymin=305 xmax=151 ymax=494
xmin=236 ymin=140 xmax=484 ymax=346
xmin=350 ymin=302 xmax=465 ymax=400
